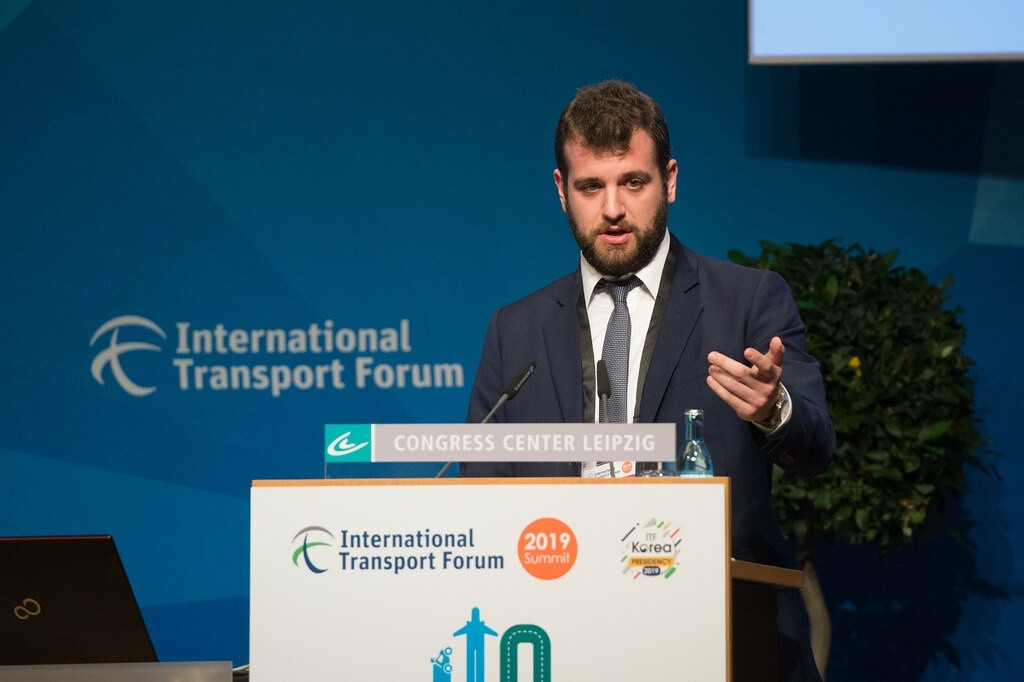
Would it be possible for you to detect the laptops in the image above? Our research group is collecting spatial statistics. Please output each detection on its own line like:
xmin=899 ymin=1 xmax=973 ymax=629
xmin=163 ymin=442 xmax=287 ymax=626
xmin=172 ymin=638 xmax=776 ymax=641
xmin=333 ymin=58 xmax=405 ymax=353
xmin=0 ymin=534 xmax=249 ymax=682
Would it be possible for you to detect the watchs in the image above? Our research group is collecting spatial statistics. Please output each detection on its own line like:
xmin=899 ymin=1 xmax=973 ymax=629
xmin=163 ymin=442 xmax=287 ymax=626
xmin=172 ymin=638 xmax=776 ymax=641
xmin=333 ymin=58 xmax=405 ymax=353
xmin=756 ymin=391 xmax=789 ymax=429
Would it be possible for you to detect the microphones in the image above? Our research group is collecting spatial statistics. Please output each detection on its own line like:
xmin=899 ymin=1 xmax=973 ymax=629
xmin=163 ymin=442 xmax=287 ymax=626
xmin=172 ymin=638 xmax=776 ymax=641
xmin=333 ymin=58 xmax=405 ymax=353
xmin=433 ymin=359 xmax=538 ymax=479
xmin=597 ymin=359 xmax=615 ymax=478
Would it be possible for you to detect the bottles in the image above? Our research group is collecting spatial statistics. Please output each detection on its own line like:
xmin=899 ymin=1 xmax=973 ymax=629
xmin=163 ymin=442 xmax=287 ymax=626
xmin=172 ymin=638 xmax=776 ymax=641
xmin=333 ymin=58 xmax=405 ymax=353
xmin=675 ymin=408 xmax=714 ymax=477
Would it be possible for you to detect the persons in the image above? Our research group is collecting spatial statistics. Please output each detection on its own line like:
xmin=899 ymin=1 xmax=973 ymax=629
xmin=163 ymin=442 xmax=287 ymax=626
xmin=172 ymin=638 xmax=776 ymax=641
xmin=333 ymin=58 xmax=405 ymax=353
xmin=460 ymin=80 xmax=836 ymax=682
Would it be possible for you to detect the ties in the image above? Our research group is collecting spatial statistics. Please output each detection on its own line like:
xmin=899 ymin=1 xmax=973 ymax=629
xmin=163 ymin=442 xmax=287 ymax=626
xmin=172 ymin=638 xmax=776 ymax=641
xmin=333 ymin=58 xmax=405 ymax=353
xmin=595 ymin=275 xmax=645 ymax=467
